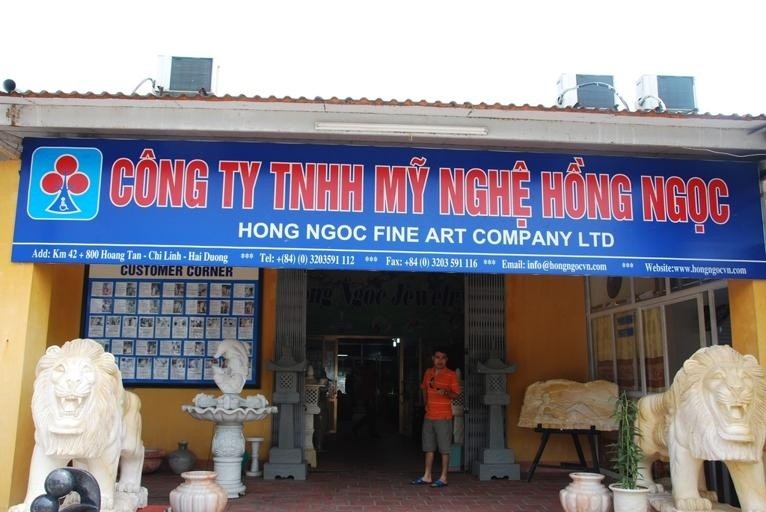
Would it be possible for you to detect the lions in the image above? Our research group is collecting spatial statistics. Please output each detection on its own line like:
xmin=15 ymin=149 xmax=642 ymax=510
xmin=630 ymin=344 xmax=766 ymax=512
xmin=7 ymin=338 xmax=145 ymax=512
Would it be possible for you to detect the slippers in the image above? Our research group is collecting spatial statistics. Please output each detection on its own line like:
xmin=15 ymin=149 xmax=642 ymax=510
xmin=408 ymin=476 xmax=448 ymax=488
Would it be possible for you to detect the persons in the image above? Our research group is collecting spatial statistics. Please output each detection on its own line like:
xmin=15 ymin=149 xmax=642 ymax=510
xmin=409 ymin=349 xmax=461 ymax=486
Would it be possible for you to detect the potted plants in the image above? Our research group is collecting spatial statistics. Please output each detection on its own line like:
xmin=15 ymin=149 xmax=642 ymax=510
xmin=608 ymin=389 xmax=651 ymax=512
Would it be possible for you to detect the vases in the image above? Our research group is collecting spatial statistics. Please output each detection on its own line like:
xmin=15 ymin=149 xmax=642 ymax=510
xmin=559 ymin=472 xmax=610 ymax=512
xmin=169 ymin=470 xmax=228 ymax=512
xmin=167 ymin=442 xmax=196 ymax=475
